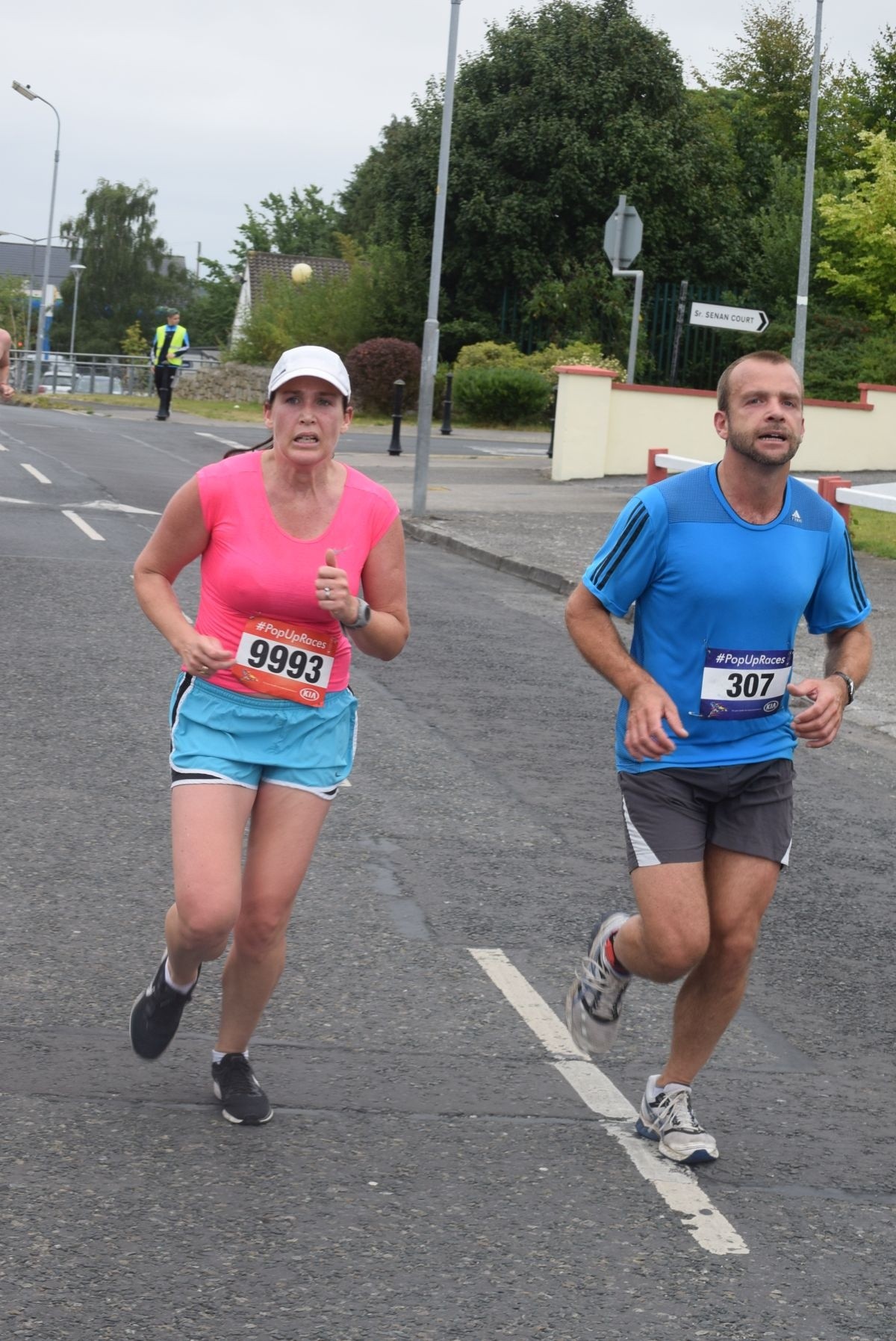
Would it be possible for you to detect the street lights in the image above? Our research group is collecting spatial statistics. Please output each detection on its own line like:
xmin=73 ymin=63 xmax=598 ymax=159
xmin=11 ymin=79 xmax=63 ymax=393
xmin=0 ymin=231 xmax=79 ymax=392
xmin=69 ymin=263 xmax=86 ymax=392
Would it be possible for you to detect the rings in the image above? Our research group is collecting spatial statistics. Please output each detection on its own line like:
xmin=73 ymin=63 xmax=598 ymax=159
xmin=200 ymin=665 xmax=208 ymax=673
xmin=324 ymin=588 xmax=329 ymax=600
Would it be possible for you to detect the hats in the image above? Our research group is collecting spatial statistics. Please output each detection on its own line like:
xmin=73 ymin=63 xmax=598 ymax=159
xmin=268 ymin=345 xmax=351 ymax=405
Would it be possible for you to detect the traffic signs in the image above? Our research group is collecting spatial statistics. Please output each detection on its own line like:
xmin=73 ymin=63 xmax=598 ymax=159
xmin=688 ymin=301 xmax=770 ymax=334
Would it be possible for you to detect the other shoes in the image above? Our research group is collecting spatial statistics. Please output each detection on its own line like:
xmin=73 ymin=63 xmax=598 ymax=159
xmin=155 ymin=414 xmax=169 ymax=420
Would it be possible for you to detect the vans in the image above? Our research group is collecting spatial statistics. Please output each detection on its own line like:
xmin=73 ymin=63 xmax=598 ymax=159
xmin=8 ymin=353 xmax=69 ymax=393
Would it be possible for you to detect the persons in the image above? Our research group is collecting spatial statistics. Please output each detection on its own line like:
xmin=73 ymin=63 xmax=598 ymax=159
xmin=565 ymin=350 xmax=875 ymax=1164
xmin=0 ymin=328 xmax=15 ymax=401
xmin=151 ymin=309 xmax=190 ymax=417
xmin=128 ymin=346 xmax=411 ymax=1124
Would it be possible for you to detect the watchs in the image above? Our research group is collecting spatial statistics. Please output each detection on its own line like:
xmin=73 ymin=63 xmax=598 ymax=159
xmin=833 ymin=671 xmax=856 ymax=704
xmin=342 ymin=598 xmax=371 ymax=628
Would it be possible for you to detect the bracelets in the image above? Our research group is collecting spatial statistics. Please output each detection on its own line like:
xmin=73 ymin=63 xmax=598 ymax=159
xmin=0 ymin=383 xmax=6 ymax=385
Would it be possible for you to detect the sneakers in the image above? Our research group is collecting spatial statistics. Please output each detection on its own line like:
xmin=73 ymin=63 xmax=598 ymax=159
xmin=566 ymin=911 xmax=633 ymax=1056
xmin=129 ymin=948 xmax=202 ymax=1060
xmin=636 ymin=1074 xmax=718 ymax=1164
xmin=209 ymin=1051 xmax=274 ymax=1125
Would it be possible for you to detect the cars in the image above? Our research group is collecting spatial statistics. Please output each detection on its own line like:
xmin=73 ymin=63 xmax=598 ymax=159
xmin=39 ymin=370 xmax=82 ymax=391
xmin=75 ymin=375 xmax=124 ymax=395
